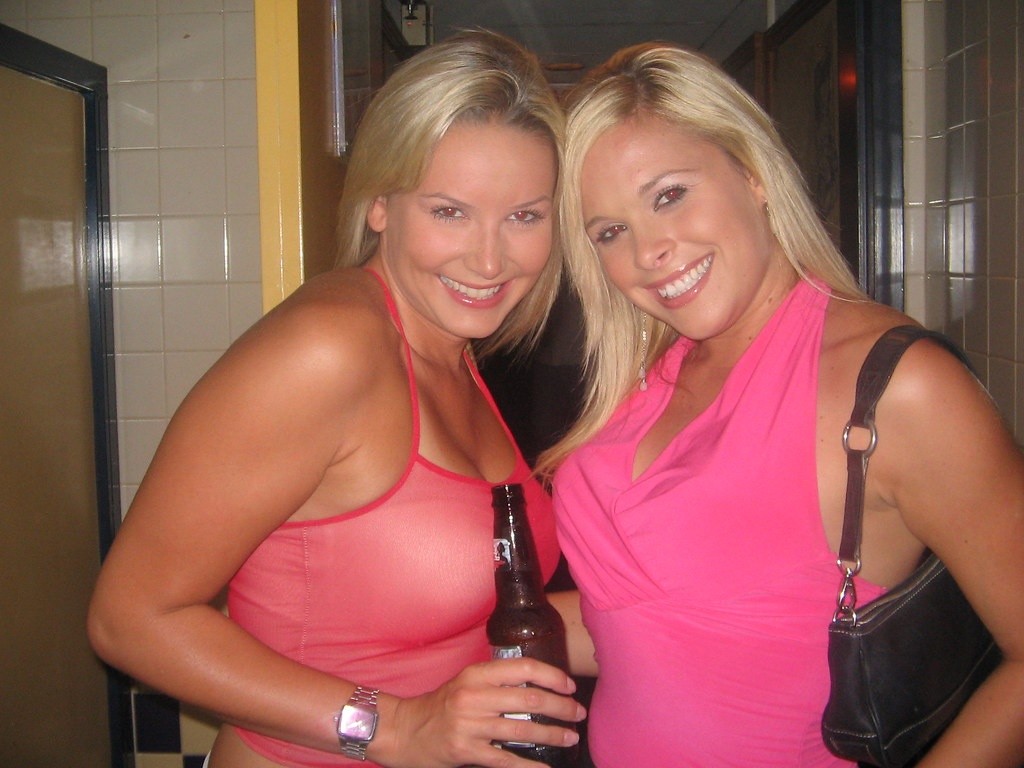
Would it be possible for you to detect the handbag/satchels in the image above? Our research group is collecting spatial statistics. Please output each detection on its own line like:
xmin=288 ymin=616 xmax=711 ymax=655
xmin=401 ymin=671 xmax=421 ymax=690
xmin=821 ymin=323 xmax=1000 ymax=768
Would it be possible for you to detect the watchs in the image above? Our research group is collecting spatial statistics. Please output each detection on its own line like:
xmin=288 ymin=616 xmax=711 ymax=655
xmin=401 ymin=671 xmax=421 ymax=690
xmin=335 ymin=685 xmax=380 ymax=762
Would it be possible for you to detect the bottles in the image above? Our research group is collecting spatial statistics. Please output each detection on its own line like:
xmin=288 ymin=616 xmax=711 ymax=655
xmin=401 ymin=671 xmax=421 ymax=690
xmin=485 ymin=482 xmax=580 ymax=768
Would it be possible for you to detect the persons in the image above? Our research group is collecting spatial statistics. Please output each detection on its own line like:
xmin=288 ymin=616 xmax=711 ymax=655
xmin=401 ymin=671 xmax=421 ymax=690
xmin=531 ymin=42 xmax=1024 ymax=767
xmin=84 ymin=27 xmax=601 ymax=768
